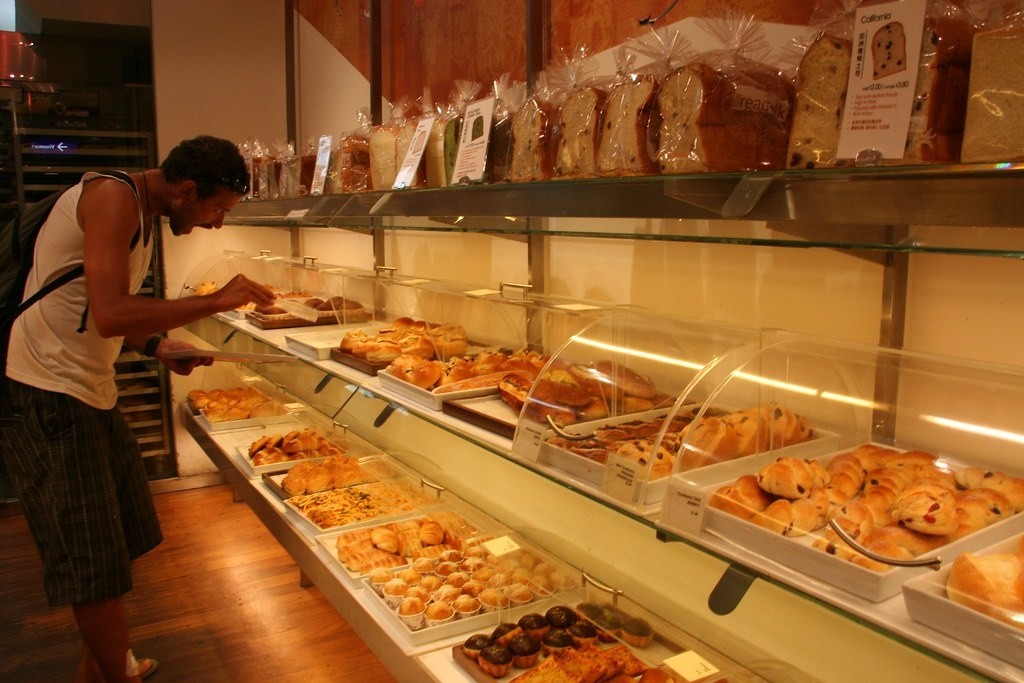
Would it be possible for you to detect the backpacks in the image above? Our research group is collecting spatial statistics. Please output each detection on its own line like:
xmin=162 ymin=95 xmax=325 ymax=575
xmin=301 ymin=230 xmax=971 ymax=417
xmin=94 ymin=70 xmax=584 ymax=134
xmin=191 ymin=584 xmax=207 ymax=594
xmin=0 ymin=170 xmax=141 ymax=353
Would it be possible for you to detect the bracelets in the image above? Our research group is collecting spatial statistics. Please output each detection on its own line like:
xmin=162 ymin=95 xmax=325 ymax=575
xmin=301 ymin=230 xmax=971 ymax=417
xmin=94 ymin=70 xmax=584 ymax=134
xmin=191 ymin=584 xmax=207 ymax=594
xmin=142 ymin=334 xmax=161 ymax=357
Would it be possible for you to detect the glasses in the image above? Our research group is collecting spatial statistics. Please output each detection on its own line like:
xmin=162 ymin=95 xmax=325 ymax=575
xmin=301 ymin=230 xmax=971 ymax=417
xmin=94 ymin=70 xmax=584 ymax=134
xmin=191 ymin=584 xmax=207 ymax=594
xmin=183 ymin=174 xmax=250 ymax=197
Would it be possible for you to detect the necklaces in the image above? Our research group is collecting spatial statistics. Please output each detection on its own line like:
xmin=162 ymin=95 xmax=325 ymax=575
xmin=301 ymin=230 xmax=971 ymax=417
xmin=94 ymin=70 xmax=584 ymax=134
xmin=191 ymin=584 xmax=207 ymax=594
xmin=142 ymin=171 xmax=158 ymax=267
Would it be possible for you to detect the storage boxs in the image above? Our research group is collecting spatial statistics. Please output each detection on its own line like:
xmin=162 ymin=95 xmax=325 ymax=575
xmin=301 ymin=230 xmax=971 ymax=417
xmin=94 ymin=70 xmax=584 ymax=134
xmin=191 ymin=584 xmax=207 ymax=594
xmin=187 ymin=291 xmax=1022 ymax=680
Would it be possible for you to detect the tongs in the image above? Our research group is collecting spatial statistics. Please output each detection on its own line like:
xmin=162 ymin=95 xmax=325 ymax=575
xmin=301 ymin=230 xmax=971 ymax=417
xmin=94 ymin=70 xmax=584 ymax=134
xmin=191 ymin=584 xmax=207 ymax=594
xmin=273 ymin=295 xmax=326 ymax=323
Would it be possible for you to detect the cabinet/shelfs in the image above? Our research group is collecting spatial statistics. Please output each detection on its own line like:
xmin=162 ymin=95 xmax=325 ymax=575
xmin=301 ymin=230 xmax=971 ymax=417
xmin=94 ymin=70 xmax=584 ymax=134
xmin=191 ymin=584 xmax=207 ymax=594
xmin=167 ymin=156 xmax=1024 ymax=683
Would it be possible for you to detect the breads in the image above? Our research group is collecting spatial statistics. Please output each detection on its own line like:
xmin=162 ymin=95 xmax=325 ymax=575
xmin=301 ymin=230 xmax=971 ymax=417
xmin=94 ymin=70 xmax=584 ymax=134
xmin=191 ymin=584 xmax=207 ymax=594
xmin=242 ymin=14 xmax=1024 ymax=204
xmin=506 ymin=645 xmax=674 ymax=683
xmin=187 ymin=281 xmax=1024 ymax=615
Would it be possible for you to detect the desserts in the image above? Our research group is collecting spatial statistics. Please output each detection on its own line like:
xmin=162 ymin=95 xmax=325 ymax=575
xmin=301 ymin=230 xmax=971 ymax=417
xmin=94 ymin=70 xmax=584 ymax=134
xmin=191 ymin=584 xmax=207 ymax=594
xmin=369 ymin=549 xmax=655 ymax=676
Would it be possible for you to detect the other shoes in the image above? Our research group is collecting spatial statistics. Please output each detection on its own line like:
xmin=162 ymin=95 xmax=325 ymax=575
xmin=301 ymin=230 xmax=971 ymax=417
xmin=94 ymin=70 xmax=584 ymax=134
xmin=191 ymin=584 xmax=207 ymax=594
xmin=138 ymin=657 xmax=159 ymax=679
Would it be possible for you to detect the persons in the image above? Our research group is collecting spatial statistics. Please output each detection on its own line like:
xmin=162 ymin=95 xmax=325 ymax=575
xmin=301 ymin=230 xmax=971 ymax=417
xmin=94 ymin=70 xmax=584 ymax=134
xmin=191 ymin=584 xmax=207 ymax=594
xmin=4 ymin=134 xmax=276 ymax=683
